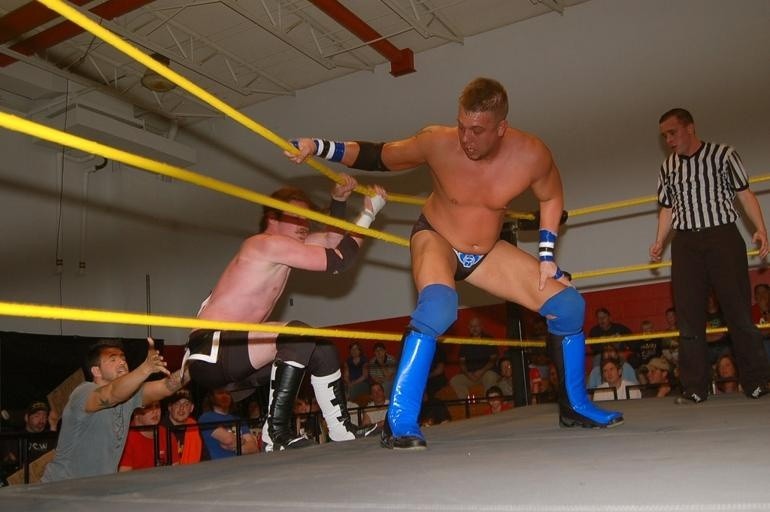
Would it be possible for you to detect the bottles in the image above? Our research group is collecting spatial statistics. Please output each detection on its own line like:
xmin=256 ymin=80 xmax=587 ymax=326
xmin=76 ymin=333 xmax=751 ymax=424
xmin=467 ymin=393 xmax=476 ymax=405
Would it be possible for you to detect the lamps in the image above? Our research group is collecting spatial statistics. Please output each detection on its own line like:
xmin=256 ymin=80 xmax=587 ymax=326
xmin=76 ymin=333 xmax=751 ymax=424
xmin=140 ymin=52 xmax=178 ymax=92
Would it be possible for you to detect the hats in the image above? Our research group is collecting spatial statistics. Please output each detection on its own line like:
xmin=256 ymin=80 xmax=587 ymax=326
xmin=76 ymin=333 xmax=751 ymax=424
xmin=643 ymin=358 xmax=670 ymax=371
xmin=27 ymin=403 xmax=47 ymax=414
xmin=168 ymin=390 xmax=192 ymax=403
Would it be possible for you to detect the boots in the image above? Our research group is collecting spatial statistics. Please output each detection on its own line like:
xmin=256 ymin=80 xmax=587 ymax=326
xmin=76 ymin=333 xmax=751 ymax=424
xmin=260 ymin=361 xmax=315 ymax=453
xmin=546 ymin=331 xmax=625 ymax=429
xmin=381 ymin=325 xmax=436 ymax=450
xmin=308 ymin=368 xmax=377 ymax=444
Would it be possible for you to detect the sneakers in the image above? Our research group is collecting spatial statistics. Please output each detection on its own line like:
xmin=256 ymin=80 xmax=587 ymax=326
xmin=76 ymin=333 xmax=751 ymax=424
xmin=744 ymin=387 xmax=770 ymax=401
xmin=675 ymin=392 xmax=707 ymax=405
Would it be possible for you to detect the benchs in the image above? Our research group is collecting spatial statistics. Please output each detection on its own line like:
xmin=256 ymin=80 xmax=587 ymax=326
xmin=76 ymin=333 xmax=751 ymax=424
xmin=434 ymin=378 xmax=492 ymax=423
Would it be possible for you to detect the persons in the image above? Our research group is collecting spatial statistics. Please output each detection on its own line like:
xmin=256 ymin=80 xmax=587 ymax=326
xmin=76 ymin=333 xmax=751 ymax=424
xmin=649 ymin=107 xmax=770 ymax=405
xmin=2 ymin=402 xmax=61 ymax=481
xmin=284 ymin=76 xmax=622 ymax=453
xmin=38 ymin=336 xmax=193 ymax=490
xmin=187 ymin=170 xmax=387 ymax=453
